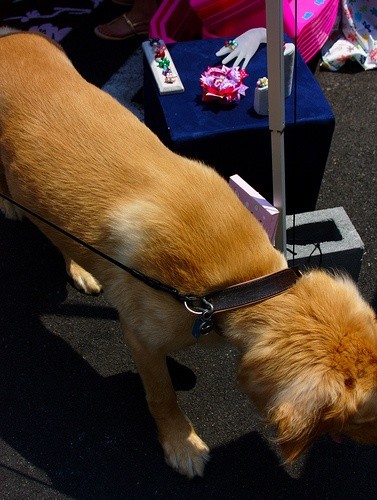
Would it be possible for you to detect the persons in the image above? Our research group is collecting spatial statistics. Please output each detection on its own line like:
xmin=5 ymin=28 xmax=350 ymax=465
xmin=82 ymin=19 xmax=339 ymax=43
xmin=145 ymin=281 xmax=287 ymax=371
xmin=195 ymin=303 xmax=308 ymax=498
xmin=95 ymin=0 xmax=163 ymax=40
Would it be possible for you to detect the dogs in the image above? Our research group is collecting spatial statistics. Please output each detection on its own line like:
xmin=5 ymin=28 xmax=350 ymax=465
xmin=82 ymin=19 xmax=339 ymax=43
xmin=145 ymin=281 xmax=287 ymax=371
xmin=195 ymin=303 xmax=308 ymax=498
xmin=0 ymin=29 xmax=377 ymax=480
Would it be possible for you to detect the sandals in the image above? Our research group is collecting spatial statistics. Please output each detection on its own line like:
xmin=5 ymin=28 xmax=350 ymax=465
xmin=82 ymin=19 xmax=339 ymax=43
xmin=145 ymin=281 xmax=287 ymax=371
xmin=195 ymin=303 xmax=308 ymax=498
xmin=94 ymin=13 xmax=150 ymax=40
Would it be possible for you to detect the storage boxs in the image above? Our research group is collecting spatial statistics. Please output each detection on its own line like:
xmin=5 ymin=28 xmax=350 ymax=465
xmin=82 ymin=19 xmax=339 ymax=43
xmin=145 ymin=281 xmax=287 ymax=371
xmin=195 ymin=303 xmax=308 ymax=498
xmin=226 ymin=176 xmax=278 ymax=247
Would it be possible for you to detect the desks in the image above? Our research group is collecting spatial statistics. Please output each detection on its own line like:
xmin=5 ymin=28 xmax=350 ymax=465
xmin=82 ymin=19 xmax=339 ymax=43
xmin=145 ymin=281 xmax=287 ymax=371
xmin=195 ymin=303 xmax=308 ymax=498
xmin=143 ymin=33 xmax=335 ymax=209
